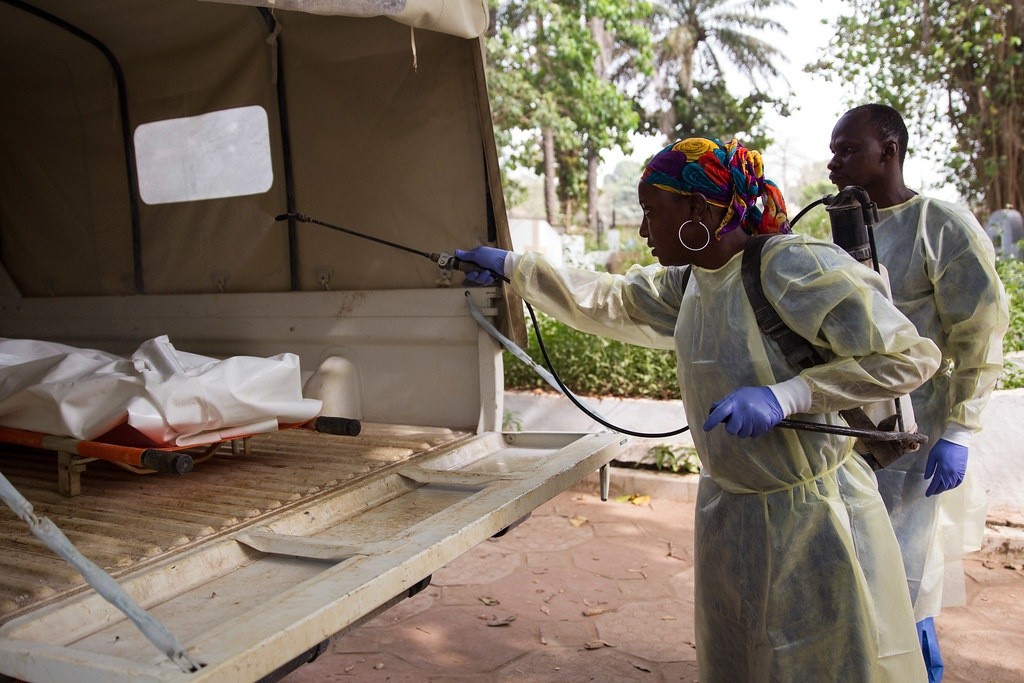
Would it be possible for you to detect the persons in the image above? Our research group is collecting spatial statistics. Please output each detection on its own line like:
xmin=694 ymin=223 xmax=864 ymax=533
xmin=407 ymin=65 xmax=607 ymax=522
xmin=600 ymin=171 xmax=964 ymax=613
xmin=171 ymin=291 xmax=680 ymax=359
xmin=824 ymin=104 xmax=1010 ymax=683
xmin=454 ymin=135 xmax=942 ymax=682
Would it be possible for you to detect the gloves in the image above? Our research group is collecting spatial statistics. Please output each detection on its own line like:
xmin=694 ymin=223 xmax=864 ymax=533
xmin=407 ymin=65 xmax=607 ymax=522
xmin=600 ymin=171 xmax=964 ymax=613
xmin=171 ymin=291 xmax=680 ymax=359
xmin=703 ymin=386 xmax=784 ymax=439
xmin=456 ymin=246 xmax=508 ymax=286
xmin=924 ymin=439 xmax=968 ymax=497
